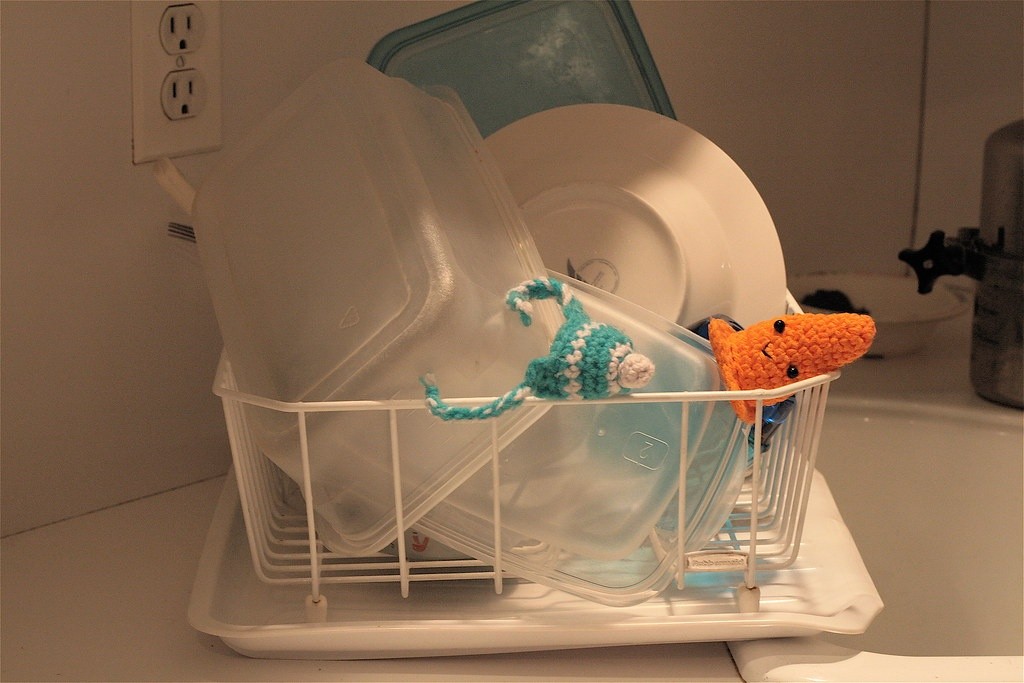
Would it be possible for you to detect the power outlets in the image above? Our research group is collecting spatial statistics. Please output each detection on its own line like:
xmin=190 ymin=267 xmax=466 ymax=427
xmin=130 ymin=0 xmax=223 ymax=166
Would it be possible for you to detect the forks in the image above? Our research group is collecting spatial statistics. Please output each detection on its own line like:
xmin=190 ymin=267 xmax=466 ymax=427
xmin=166 ymin=220 xmax=198 ymax=244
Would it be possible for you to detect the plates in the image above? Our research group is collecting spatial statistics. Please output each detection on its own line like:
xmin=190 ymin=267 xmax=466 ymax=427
xmin=479 ymin=102 xmax=787 ymax=342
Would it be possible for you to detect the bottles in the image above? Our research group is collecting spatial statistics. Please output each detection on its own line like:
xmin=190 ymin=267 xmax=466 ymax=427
xmin=972 ymin=118 xmax=1024 ymax=411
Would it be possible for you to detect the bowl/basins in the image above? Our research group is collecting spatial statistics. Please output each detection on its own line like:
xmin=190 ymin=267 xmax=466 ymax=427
xmin=192 ymin=54 xmax=568 ymax=559
xmin=787 ymin=272 xmax=974 ymax=361
